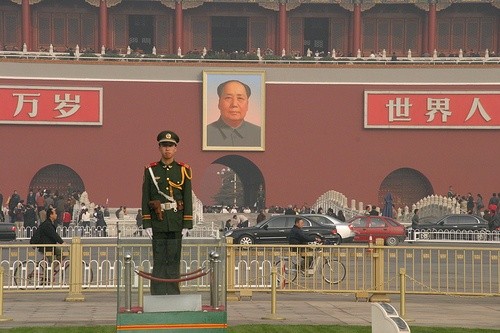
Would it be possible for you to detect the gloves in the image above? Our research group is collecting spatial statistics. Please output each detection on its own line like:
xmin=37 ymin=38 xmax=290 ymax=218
xmin=181 ymin=228 xmax=189 ymax=240
xmin=146 ymin=228 xmax=152 ymax=239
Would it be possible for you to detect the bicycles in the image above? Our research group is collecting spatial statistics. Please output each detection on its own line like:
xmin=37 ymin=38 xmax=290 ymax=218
xmin=13 ymin=247 xmax=94 ymax=290
xmin=274 ymin=241 xmax=346 ymax=285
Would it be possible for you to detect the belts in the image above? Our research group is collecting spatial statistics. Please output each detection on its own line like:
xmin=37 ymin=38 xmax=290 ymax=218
xmin=151 ymin=203 xmax=178 ymax=212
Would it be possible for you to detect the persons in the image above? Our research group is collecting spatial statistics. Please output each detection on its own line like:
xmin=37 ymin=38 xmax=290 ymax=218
xmin=191 ymin=48 xmax=376 ymax=58
xmin=0 ymin=189 xmax=128 ymax=237
xmin=204 ymin=204 xmax=345 ymax=227
xmin=287 ymin=217 xmax=321 ymax=277
xmin=411 ymin=209 xmax=421 ymax=240
xmin=142 ymin=130 xmax=193 ymax=295
xmin=364 ymin=204 xmax=397 ymax=220
xmin=447 ymin=185 xmax=500 ymax=241
xmin=4 ymin=44 xmax=144 ymax=53
xmin=36 ymin=208 xmax=66 ymax=285
xmin=421 ymin=49 xmax=496 ymax=57
xmin=207 ymin=80 xmax=261 ymax=146
xmin=392 ymin=52 xmax=396 ymax=60
xmin=133 ymin=209 xmax=143 ymax=237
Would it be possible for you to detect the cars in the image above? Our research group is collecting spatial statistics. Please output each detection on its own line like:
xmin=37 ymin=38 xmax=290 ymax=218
xmin=223 ymin=215 xmax=340 ymax=251
xmin=301 ymin=214 xmax=355 ymax=245
xmin=404 ymin=214 xmax=489 ymax=241
xmin=0 ymin=221 xmax=17 ymax=242
xmin=345 ymin=214 xmax=407 ymax=246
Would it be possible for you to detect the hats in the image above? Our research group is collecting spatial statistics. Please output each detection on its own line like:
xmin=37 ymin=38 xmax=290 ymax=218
xmin=157 ymin=130 xmax=179 ymax=146
